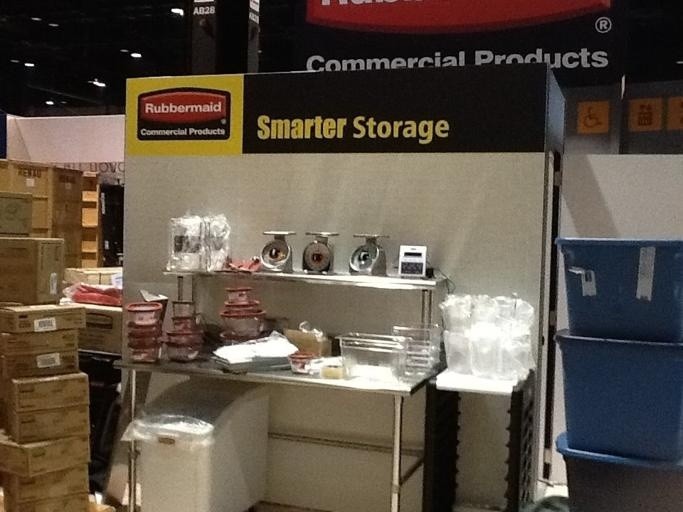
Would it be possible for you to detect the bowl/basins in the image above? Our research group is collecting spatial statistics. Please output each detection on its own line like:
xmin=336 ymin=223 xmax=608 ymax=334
xmin=221 ymin=310 xmax=267 ymax=337
xmin=125 ymin=301 xmax=203 ymax=366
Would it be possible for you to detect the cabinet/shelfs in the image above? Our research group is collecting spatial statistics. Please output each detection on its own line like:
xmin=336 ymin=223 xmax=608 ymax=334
xmin=116 ymin=268 xmax=447 ymax=511
xmin=80 ymin=170 xmax=102 ymax=266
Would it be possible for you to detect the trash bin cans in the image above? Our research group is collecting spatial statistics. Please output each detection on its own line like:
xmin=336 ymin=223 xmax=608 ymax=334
xmin=135 ymin=378 xmax=272 ymax=512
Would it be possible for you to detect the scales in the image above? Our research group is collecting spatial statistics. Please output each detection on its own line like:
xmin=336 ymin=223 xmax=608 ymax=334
xmin=260 ymin=231 xmax=390 ymax=277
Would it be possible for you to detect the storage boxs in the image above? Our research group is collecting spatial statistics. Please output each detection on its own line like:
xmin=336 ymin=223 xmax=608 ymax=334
xmin=60 ymin=300 xmax=121 ymax=352
xmin=2 ymin=493 xmax=94 ymax=511
xmin=1 ymin=303 xmax=86 ymax=333
xmin=555 ymin=237 xmax=683 ymax=512
xmin=1 ymin=373 xmax=89 ymax=412
xmin=2 ymin=329 xmax=78 ymax=356
xmin=2 ymin=236 xmax=64 ymax=302
xmin=1 ymin=160 xmax=80 ymax=271
xmin=66 ymin=266 xmax=121 ymax=286
xmin=3 ymin=465 xmax=90 ymax=502
xmin=1 ymin=437 xmax=91 ymax=476
xmin=1 ymin=191 xmax=32 ymax=235
xmin=5 ymin=406 xmax=91 ymax=443
xmin=2 ymin=352 xmax=79 ymax=379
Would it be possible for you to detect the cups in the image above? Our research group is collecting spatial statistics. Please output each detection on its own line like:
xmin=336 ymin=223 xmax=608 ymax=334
xmin=288 ymin=353 xmax=312 ymax=375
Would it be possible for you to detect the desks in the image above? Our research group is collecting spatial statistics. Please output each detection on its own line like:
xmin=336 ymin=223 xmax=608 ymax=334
xmin=77 ymin=349 xmax=120 ymax=470
